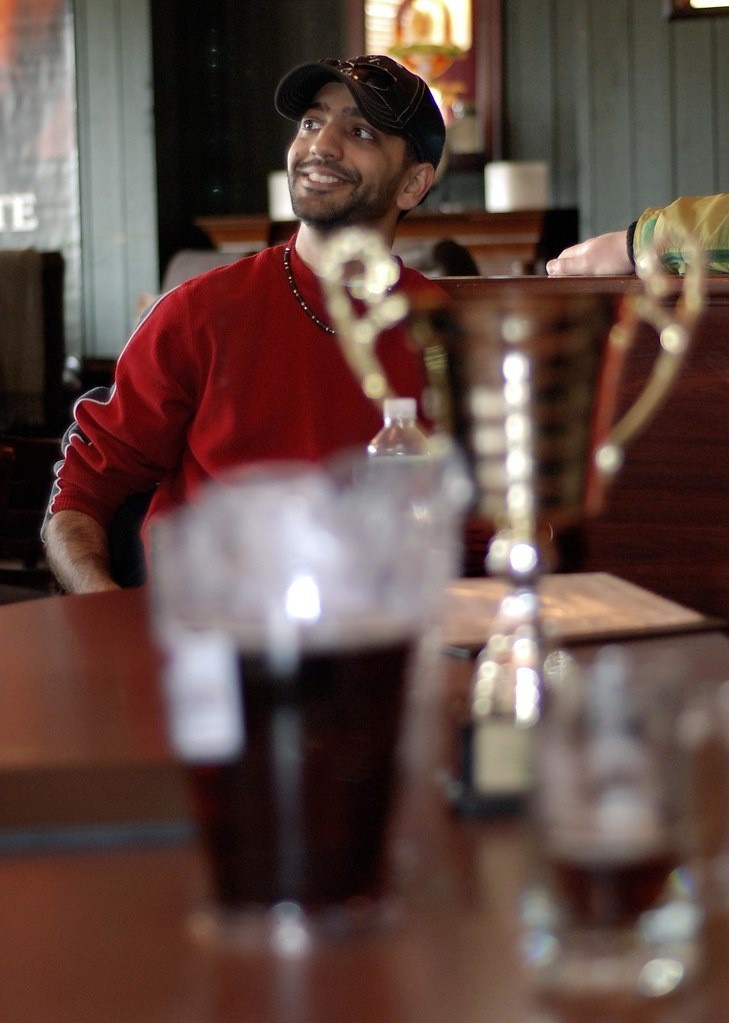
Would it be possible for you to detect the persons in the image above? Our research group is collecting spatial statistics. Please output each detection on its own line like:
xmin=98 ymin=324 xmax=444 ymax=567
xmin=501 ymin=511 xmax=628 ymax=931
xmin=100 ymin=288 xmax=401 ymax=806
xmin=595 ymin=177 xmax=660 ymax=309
xmin=546 ymin=193 xmax=729 ymax=276
xmin=42 ymin=56 xmax=453 ymax=594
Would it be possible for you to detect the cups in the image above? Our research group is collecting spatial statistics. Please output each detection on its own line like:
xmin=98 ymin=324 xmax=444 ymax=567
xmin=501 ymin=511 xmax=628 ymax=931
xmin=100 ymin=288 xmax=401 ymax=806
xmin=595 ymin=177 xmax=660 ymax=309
xmin=484 ymin=160 xmax=552 ymax=213
xmin=267 ymin=171 xmax=301 ymax=222
xmin=509 ymin=644 xmax=712 ymax=1001
xmin=153 ymin=465 xmax=449 ymax=967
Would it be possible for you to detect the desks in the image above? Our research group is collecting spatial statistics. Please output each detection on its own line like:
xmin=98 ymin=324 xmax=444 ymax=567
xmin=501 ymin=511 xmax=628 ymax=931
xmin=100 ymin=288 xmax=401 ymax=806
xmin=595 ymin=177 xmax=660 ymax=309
xmin=0 ymin=573 xmax=729 ymax=1023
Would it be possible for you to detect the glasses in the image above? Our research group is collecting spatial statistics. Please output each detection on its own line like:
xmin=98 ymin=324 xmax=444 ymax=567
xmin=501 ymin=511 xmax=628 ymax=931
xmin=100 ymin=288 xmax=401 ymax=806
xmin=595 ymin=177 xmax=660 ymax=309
xmin=317 ymin=56 xmax=437 ymax=172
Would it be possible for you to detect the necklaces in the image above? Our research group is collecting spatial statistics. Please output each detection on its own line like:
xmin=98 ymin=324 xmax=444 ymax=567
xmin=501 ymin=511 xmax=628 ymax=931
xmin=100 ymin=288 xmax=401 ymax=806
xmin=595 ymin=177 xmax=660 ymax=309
xmin=284 ymin=243 xmax=392 ymax=336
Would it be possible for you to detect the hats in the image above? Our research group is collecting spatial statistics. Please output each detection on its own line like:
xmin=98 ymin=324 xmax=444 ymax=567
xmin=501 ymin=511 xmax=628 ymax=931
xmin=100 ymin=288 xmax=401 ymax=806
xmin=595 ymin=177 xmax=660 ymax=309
xmin=274 ymin=54 xmax=448 ymax=172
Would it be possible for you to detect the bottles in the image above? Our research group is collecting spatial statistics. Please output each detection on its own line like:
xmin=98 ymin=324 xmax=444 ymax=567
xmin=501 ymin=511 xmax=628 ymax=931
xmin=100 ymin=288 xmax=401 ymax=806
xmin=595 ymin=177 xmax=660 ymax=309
xmin=365 ymin=397 xmax=432 ymax=459
